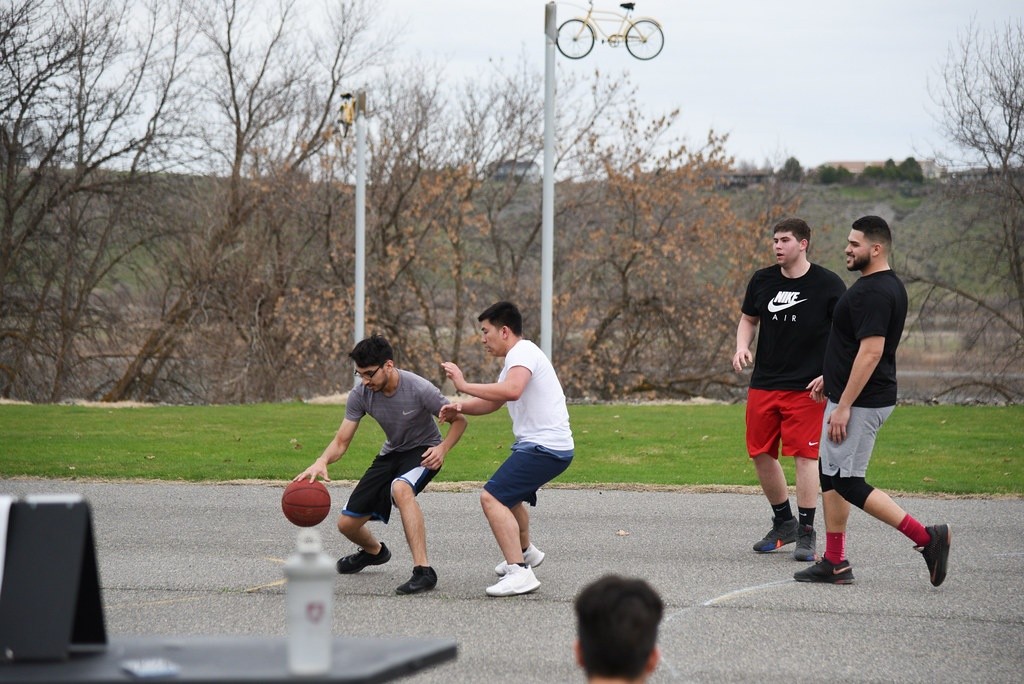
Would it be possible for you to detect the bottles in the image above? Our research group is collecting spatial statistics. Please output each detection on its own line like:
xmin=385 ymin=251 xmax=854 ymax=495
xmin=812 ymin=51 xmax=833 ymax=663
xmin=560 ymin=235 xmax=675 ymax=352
xmin=282 ymin=529 xmax=337 ymax=676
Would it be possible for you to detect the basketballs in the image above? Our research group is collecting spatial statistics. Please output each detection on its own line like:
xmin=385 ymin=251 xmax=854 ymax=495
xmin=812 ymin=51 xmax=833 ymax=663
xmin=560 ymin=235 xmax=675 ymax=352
xmin=281 ymin=478 xmax=331 ymax=528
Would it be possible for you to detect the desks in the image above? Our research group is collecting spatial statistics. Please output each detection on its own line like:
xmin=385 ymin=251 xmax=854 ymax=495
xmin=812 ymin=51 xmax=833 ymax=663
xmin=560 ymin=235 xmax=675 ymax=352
xmin=0 ymin=634 xmax=459 ymax=684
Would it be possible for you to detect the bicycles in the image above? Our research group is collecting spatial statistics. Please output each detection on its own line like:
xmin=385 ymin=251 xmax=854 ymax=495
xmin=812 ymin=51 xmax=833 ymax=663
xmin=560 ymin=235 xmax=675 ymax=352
xmin=557 ymin=2 xmax=666 ymax=60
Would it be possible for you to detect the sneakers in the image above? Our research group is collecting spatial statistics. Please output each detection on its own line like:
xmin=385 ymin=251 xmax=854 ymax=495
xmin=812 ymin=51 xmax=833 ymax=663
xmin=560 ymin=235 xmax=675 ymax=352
xmin=794 ymin=553 xmax=856 ymax=585
xmin=794 ymin=524 xmax=817 ymax=561
xmin=336 ymin=541 xmax=391 ymax=574
xmin=912 ymin=523 xmax=951 ymax=587
xmin=495 ymin=543 xmax=545 ymax=575
xmin=395 ymin=566 xmax=438 ymax=595
xmin=753 ymin=515 xmax=801 ymax=551
xmin=486 ymin=563 xmax=540 ymax=596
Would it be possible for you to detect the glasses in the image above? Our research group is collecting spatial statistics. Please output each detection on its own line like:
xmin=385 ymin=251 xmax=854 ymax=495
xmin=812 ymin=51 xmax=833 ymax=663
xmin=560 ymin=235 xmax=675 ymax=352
xmin=354 ymin=361 xmax=386 ymax=379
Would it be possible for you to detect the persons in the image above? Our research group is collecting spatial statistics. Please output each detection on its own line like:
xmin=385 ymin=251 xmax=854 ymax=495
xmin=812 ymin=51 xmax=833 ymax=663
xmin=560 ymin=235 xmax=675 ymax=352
xmin=437 ymin=300 xmax=574 ymax=596
xmin=572 ymin=572 xmax=665 ymax=684
xmin=732 ymin=217 xmax=847 ymax=560
xmin=793 ymin=216 xmax=951 ymax=586
xmin=291 ymin=336 xmax=467 ymax=594
